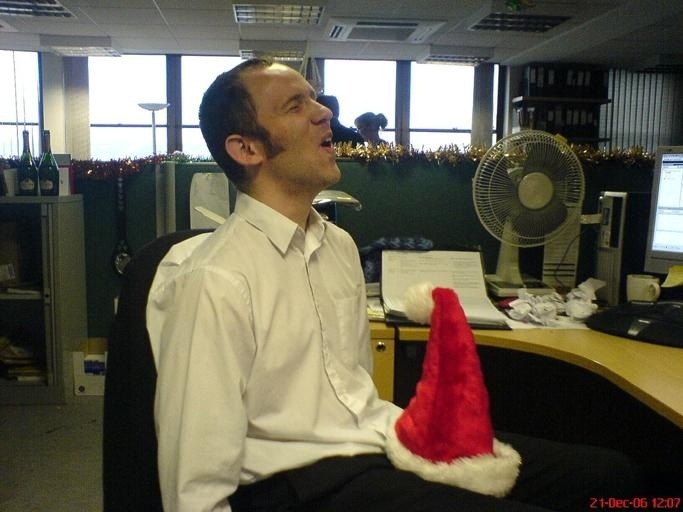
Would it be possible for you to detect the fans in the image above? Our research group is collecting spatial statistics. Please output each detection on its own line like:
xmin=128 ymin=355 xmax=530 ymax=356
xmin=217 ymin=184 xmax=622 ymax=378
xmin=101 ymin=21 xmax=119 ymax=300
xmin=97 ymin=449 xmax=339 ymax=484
xmin=472 ymin=130 xmax=586 ymax=297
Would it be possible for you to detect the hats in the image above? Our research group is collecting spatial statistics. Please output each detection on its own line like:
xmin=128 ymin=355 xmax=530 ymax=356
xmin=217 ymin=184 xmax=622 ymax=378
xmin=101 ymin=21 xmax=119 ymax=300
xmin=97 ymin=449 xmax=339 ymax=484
xmin=383 ymin=285 xmax=523 ymax=501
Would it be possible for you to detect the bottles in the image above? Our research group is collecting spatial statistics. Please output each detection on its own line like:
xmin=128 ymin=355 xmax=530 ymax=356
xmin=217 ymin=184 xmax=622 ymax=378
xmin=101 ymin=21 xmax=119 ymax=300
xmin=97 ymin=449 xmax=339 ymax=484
xmin=17 ymin=129 xmax=58 ymax=196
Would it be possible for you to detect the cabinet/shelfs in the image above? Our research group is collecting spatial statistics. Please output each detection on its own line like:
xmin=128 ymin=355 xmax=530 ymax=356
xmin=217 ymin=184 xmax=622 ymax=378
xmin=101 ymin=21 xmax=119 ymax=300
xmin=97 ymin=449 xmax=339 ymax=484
xmin=1 ymin=194 xmax=88 ymax=405
xmin=511 ymin=61 xmax=615 ymax=156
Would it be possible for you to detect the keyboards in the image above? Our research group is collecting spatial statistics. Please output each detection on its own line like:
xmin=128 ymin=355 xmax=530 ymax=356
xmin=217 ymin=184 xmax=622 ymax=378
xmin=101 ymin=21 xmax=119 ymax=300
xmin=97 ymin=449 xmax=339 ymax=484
xmin=585 ymin=299 xmax=683 ymax=349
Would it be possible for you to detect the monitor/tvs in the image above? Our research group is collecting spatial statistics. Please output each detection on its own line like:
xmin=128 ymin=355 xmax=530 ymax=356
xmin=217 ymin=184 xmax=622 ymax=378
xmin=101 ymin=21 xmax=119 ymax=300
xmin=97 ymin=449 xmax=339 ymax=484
xmin=643 ymin=144 xmax=683 ymax=275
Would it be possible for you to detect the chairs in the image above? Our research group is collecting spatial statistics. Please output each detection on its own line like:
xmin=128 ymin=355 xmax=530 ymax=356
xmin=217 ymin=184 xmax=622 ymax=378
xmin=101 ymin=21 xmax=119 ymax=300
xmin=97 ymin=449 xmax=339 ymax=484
xmin=102 ymin=229 xmax=216 ymax=512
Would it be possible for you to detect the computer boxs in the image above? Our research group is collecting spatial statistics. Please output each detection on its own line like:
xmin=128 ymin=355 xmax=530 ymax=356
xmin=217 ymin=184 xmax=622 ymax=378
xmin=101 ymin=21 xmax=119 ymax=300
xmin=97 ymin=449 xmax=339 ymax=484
xmin=594 ymin=190 xmax=652 ymax=306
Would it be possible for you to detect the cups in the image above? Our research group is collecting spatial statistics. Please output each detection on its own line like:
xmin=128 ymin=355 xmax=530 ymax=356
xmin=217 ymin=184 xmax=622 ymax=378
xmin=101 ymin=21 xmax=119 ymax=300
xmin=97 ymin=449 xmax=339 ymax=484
xmin=3 ymin=169 xmax=17 ymax=197
xmin=627 ymin=273 xmax=660 ymax=305
xmin=58 ymin=168 xmax=69 ymax=196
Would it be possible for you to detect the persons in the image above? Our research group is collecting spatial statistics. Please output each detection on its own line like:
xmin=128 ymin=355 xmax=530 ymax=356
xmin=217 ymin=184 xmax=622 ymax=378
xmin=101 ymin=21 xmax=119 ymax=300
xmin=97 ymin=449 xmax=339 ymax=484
xmin=353 ymin=110 xmax=390 ymax=145
xmin=144 ymin=54 xmax=639 ymax=512
xmin=315 ymin=93 xmax=363 ymax=148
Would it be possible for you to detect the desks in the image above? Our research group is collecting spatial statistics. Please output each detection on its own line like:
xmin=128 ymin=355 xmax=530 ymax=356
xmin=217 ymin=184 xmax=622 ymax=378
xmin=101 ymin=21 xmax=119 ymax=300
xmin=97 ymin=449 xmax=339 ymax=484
xmin=367 ymin=297 xmax=683 ymax=430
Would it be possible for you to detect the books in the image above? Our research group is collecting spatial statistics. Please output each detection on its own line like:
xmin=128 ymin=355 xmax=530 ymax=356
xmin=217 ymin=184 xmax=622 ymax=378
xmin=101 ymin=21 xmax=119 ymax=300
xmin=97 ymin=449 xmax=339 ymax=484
xmin=7 ymin=283 xmax=42 ymax=294
xmin=0 ymin=336 xmax=47 ymax=384
xmin=511 ymin=60 xmax=608 ymax=139
xmin=377 ymin=246 xmax=512 ymax=335
